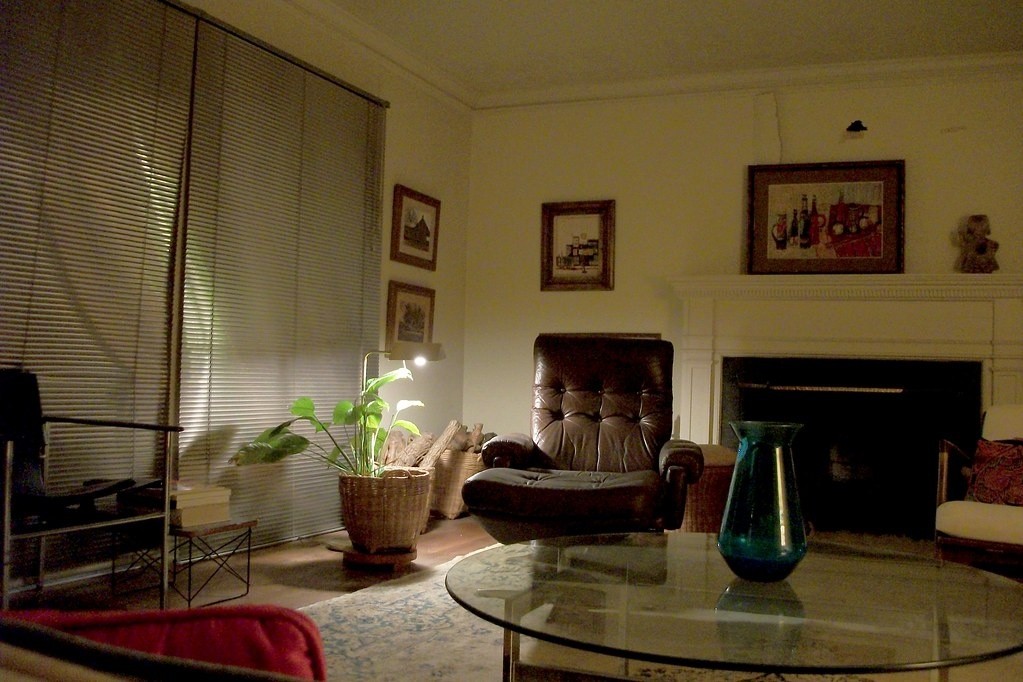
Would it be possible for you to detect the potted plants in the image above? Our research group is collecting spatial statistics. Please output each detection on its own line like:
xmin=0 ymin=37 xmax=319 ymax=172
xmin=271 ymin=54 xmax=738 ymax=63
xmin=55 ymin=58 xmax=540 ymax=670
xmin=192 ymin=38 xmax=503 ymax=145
xmin=228 ymin=361 xmax=430 ymax=553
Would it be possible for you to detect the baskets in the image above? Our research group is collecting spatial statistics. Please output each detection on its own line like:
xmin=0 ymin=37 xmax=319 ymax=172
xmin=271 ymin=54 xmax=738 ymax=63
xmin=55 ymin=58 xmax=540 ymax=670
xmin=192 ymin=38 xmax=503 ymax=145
xmin=669 ymin=443 xmax=736 ymax=533
xmin=431 ymin=448 xmax=487 ymax=520
xmin=418 ymin=466 xmax=435 ymax=536
xmin=338 ymin=460 xmax=431 ymax=555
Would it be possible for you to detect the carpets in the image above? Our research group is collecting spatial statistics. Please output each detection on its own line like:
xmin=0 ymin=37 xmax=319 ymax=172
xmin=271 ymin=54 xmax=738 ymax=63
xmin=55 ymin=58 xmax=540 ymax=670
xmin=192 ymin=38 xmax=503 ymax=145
xmin=293 ymin=538 xmax=1023 ymax=682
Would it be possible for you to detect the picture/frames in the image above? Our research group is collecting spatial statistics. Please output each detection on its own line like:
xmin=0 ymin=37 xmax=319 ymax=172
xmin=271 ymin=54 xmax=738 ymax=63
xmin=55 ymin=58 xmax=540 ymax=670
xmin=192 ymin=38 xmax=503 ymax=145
xmin=384 ymin=279 xmax=437 ymax=359
xmin=747 ymin=159 xmax=905 ymax=275
xmin=390 ymin=182 xmax=441 ymax=272
xmin=540 ymin=200 xmax=616 ymax=291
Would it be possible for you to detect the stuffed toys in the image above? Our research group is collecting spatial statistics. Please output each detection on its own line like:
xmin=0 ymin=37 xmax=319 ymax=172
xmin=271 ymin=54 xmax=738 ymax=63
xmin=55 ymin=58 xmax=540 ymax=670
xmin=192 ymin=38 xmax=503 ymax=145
xmin=959 ymin=213 xmax=999 ymax=274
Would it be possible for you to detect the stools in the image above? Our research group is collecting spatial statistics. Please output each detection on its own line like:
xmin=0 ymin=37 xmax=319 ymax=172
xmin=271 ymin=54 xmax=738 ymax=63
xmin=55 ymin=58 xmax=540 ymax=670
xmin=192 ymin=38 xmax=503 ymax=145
xmin=676 ymin=441 xmax=737 ymax=532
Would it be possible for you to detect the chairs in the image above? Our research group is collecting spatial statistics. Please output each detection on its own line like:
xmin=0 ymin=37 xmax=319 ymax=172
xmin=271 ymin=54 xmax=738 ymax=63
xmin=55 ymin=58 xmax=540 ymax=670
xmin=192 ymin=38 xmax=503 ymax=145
xmin=935 ymin=404 xmax=1023 ymax=560
xmin=0 ymin=369 xmax=184 ymax=611
xmin=462 ymin=332 xmax=704 ymax=545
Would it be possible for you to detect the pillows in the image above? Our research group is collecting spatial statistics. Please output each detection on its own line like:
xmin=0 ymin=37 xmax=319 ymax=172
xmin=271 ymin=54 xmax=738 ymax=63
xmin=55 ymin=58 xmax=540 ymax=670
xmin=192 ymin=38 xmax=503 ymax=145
xmin=964 ymin=438 xmax=1023 ymax=506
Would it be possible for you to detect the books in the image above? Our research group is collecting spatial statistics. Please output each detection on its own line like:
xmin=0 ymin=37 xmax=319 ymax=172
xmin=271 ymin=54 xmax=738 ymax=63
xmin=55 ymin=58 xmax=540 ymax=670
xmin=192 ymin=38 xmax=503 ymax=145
xmin=117 ymin=479 xmax=232 ymax=527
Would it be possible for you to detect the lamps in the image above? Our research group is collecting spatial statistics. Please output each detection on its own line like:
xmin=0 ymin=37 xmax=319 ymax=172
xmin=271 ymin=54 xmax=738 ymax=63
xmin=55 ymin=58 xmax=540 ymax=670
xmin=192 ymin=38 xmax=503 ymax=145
xmin=362 ymin=340 xmax=446 ymax=400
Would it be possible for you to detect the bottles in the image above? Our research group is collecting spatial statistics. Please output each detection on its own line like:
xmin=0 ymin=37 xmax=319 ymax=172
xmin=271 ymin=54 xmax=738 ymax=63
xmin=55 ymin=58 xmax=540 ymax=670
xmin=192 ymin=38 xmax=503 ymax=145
xmin=716 ymin=419 xmax=810 ymax=584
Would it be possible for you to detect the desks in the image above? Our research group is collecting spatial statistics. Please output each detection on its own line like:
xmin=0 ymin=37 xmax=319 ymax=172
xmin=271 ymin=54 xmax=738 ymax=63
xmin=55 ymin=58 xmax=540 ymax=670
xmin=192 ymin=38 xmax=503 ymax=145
xmin=445 ymin=531 xmax=1023 ymax=682
xmin=93 ymin=505 xmax=257 ymax=610
